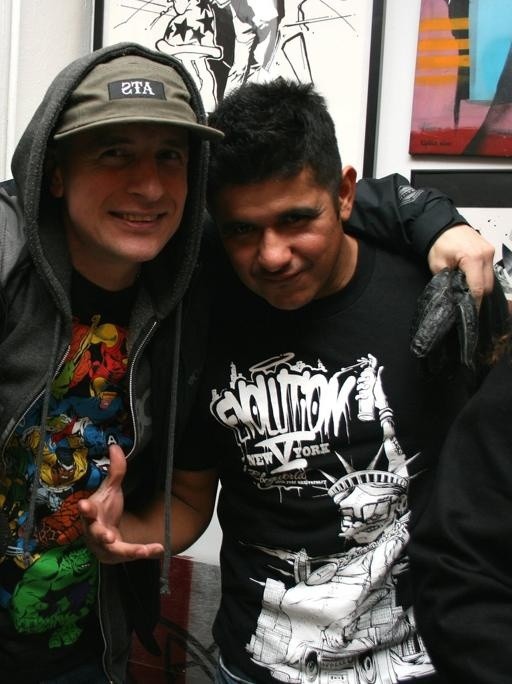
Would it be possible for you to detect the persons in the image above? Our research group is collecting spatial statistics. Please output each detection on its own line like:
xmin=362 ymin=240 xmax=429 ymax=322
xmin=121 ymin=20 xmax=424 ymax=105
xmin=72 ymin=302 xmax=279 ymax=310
xmin=402 ymin=346 xmax=512 ymax=684
xmin=78 ymin=75 xmax=506 ymax=684
xmin=0 ymin=39 xmax=496 ymax=684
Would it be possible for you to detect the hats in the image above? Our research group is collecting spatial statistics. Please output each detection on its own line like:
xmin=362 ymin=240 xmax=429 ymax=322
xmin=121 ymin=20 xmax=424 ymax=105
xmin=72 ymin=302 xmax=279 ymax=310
xmin=48 ymin=50 xmax=226 ymax=141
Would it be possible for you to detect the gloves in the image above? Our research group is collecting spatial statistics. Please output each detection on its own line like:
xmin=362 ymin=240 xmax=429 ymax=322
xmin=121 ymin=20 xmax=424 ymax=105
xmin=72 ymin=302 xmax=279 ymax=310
xmin=409 ymin=261 xmax=511 ymax=374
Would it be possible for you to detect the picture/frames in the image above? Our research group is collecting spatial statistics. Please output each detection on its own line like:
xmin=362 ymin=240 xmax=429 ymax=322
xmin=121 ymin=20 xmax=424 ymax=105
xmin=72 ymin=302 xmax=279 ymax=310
xmin=92 ymin=0 xmax=386 ymax=182
xmin=408 ymin=168 xmax=511 ymax=314
